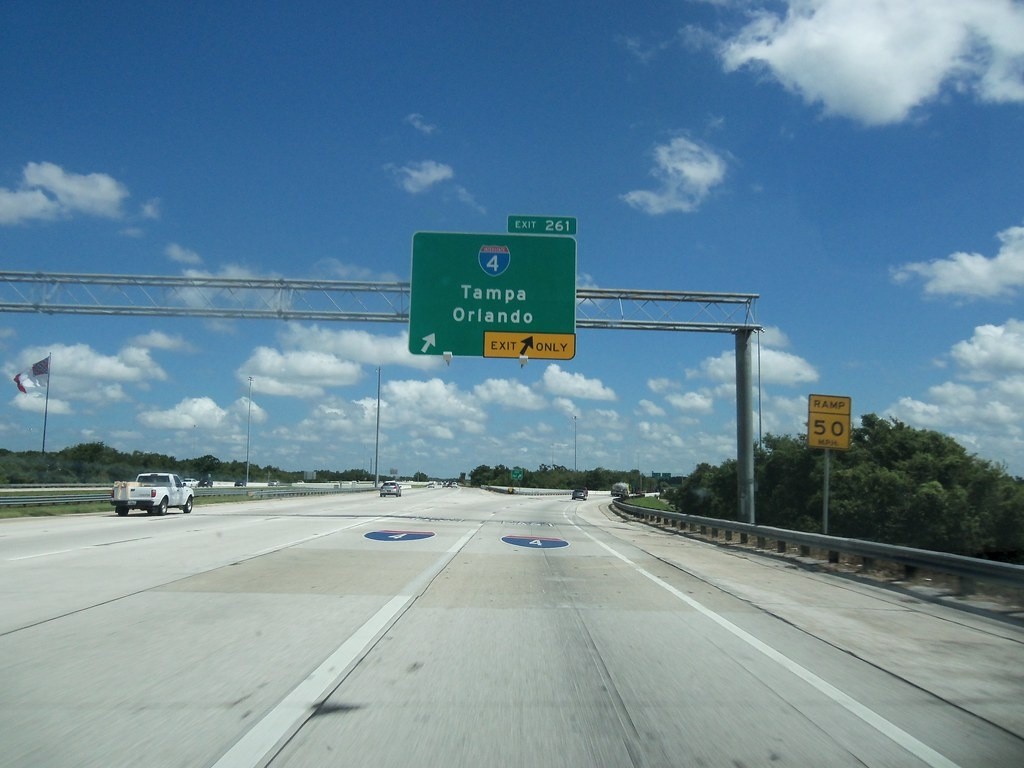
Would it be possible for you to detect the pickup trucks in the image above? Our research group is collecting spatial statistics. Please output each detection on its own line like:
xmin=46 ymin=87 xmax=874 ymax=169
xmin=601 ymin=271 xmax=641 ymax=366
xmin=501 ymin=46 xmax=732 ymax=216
xmin=111 ymin=472 xmax=195 ymax=516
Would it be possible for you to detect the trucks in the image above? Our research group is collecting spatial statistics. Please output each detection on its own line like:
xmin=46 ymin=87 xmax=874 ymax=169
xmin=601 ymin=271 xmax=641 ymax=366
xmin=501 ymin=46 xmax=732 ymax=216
xmin=611 ymin=482 xmax=630 ymax=498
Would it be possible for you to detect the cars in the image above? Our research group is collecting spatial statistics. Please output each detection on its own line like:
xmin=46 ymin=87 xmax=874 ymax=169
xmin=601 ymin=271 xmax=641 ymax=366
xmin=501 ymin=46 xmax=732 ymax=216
xmin=379 ymin=481 xmax=402 ymax=497
xmin=197 ymin=478 xmax=214 ymax=488
xmin=268 ymin=479 xmax=281 ymax=487
xmin=572 ymin=488 xmax=588 ymax=501
xmin=180 ymin=477 xmax=200 ymax=489
xmin=235 ymin=480 xmax=246 ymax=488
xmin=428 ymin=480 xmax=458 ymax=489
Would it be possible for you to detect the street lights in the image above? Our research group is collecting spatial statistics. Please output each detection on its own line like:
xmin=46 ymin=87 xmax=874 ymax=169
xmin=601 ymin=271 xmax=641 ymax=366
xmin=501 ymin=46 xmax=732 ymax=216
xmin=375 ymin=363 xmax=382 ymax=488
xmin=247 ymin=376 xmax=255 ymax=488
xmin=573 ymin=415 xmax=577 ymax=470
